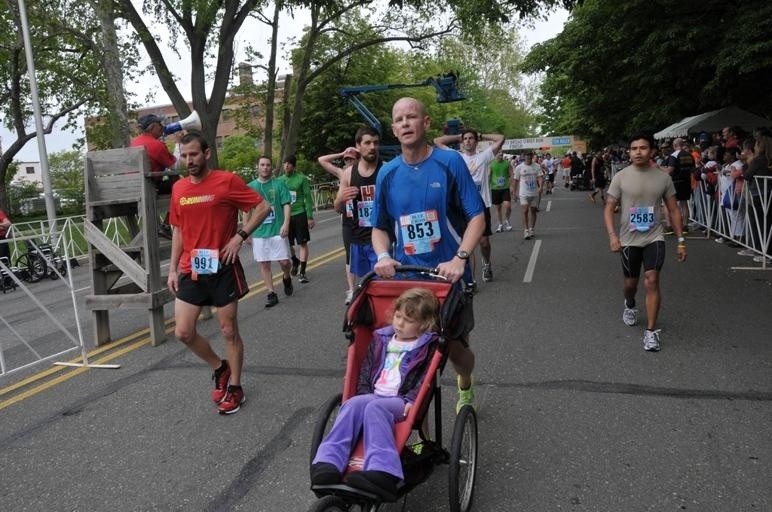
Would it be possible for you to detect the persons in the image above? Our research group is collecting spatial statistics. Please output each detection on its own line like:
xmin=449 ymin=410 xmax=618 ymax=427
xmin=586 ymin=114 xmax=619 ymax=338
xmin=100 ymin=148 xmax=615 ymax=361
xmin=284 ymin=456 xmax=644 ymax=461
xmin=652 ymin=125 xmax=770 ymax=264
xmin=242 ymin=156 xmax=297 ymax=307
xmin=433 ymin=128 xmax=504 ymax=283
xmin=318 ymin=126 xmax=389 ymax=307
xmin=604 ymin=132 xmax=686 ymax=350
xmin=372 ymin=97 xmax=486 ymax=416
xmin=167 ymin=132 xmax=273 ymax=415
xmin=560 ymin=146 xmax=633 ymax=205
xmin=0 ymin=207 xmax=19 ymax=290
xmin=312 ymin=289 xmax=441 ymax=496
xmin=126 ymin=115 xmax=187 ymax=240
xmin=276 ymin=154 xmax=315 ymax=284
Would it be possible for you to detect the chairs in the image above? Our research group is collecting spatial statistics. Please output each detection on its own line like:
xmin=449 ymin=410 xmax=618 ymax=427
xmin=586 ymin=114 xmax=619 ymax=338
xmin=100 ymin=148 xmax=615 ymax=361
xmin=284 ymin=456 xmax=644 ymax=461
xmin=78 ymin=143 xmax=214 ymax=346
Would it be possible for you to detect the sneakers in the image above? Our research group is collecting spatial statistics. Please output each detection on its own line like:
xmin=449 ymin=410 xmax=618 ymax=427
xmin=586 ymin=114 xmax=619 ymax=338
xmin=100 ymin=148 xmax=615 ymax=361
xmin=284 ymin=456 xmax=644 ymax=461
xmin=644 ymin=329 xmax=662 ymax=351
xmin=345 ymin=285 xmax=359 ymax=305
xmin=454 ymin=373 xmax=476 ymax=420
xmin=623 ymin=300 xmax=640 ymax=325
xmin=290 ymin=259 xmax=300 ymax=276
xmin=343 ymin=470 xmax=400 ymax=493
xmin=658 ymin=220 xmax=702 ymax=235
xmin=282 ymin=274 xmax=293 ymax=297
xmin=211 ymin=358 xmax=231 ymax=403
xmin=310 ymin=463 xmax=340 ymax=493
xmin=264 ymin=291 xmax=280 ymax=307
xmin=298 ymin=273 xmax=309 ymax=283
xmin=524 ymin=230 xmax=530 ymax=239
xmin=701 ymin=227 xmax=772 ymax=264
xmin=546 ymin=189 xmax=549 ymax=194
xmin=217 ymin=386 xmax=246 ymax=414
xmin=599 ymin=196 xmax=606 ymax=204
xmin=588 ymin=193 xmax=596 ymax=202
xmin=550 ymin=190 xmax=553 ymax=194
xmin=496 ymin=224 xmax=504 ymax=232
xmin=529 ymin=228 xmax=535 ymax=236
xmin=504 ymin=219 xmax=513 ymax=231
xmin=482 ymin=257 xmax=493 ymax=282
xmin=158 ymin=222 xmax=173 ymax=241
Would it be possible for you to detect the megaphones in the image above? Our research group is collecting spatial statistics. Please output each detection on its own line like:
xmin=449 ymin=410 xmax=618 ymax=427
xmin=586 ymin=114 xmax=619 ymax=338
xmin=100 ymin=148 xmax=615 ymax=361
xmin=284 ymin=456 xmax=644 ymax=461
xmin=161 ymin=110 xmax=205 ymax=133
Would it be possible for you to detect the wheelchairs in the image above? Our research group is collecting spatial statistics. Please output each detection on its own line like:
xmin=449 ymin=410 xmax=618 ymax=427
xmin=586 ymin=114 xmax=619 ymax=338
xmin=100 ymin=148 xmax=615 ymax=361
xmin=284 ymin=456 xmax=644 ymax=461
xmin=14 ymin=239 xmax=80 ymax=283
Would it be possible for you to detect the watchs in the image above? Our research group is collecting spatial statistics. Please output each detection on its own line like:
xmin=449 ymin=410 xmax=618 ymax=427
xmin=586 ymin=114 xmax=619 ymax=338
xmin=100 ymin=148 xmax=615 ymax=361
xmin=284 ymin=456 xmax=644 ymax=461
xmin=455 ymin=250 xmax=470 ymax=262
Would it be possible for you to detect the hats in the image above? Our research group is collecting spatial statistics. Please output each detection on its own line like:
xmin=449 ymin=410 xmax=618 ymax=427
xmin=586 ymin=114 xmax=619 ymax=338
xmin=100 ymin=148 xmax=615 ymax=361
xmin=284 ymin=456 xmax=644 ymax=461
xmin=342 ymin=148 xmax=357 ymax=161
xmin=136 ymin=113 xmax=164 ymax=130
xmin=524 ymin=149 xmax=533 ymax=156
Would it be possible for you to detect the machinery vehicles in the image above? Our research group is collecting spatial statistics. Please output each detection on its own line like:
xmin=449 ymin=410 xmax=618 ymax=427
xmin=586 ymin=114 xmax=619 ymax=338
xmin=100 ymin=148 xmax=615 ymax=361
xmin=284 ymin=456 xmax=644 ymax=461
xmin=338 ymin=70 xmax=467 ymax=162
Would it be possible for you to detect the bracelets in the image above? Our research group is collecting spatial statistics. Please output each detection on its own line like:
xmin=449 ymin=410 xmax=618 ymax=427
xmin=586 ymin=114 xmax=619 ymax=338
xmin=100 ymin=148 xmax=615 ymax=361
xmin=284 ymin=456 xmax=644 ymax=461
xmin=237 ymin=229 xmax=250 ymax=241
xmin=377 ymin=251 xmax=390 ymax=260
xmin=479 ymin=133 xmax=482 ymax=140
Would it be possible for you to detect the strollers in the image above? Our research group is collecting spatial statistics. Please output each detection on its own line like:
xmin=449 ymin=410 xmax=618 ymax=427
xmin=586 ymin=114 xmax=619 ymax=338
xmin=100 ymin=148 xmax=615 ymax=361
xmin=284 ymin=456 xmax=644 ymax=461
xmin=306 ymin=264 xmax=479 ymax=510
